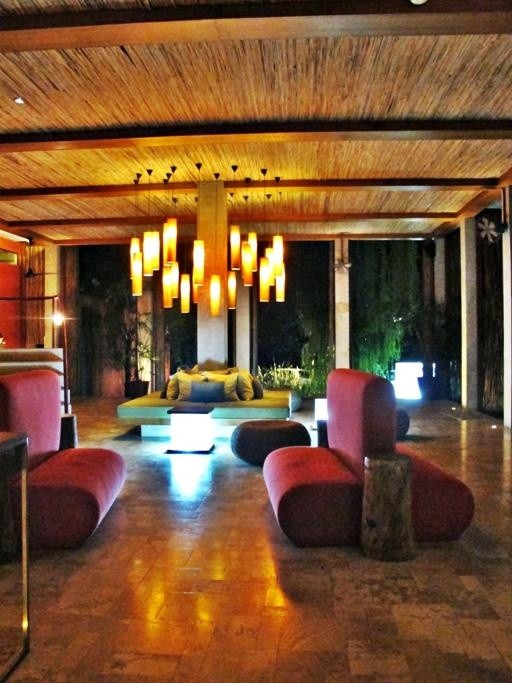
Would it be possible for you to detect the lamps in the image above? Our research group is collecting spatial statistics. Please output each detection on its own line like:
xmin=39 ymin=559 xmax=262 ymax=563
xmin=50 ymin=296 xmax=69 ymax=417
xmin=129 ymin=163 xmax=286 ymax=316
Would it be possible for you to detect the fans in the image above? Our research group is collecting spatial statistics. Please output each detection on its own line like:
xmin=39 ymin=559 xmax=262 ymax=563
xmin=22 ymin=236 xmax=61 ymax=278
xmin=474 ymin=210 xmax=507 ymax=244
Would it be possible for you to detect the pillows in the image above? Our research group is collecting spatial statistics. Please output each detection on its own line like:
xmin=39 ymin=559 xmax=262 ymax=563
xmin=160 ymin=358 xmax=263 ymax=401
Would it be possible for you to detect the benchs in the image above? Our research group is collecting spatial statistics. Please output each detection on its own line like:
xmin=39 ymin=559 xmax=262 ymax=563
xmin=117 ymin=382 xmax=291 ymax=437
xmin=0 ymin=367 xmax=125 ymax=549
xmin=262 ymin=369 xmax=474 ymax=546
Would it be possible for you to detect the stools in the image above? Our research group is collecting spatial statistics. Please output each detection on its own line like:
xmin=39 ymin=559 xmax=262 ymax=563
xmin=396 ymin=404 xmax=410 ymax=439
xmin=60 ymin=416 xmax=77 ymax=449
xmin=231 ymin=419 xmax=311 ymax=466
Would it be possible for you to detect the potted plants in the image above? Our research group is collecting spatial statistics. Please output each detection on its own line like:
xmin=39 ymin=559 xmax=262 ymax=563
xmin=80 ymin=260 xmax=156 ymax=398
xmin=257 ymin=362 xmax=311 ymax=412
xmin=401 ymin=297 xmax=461 ymax=399
